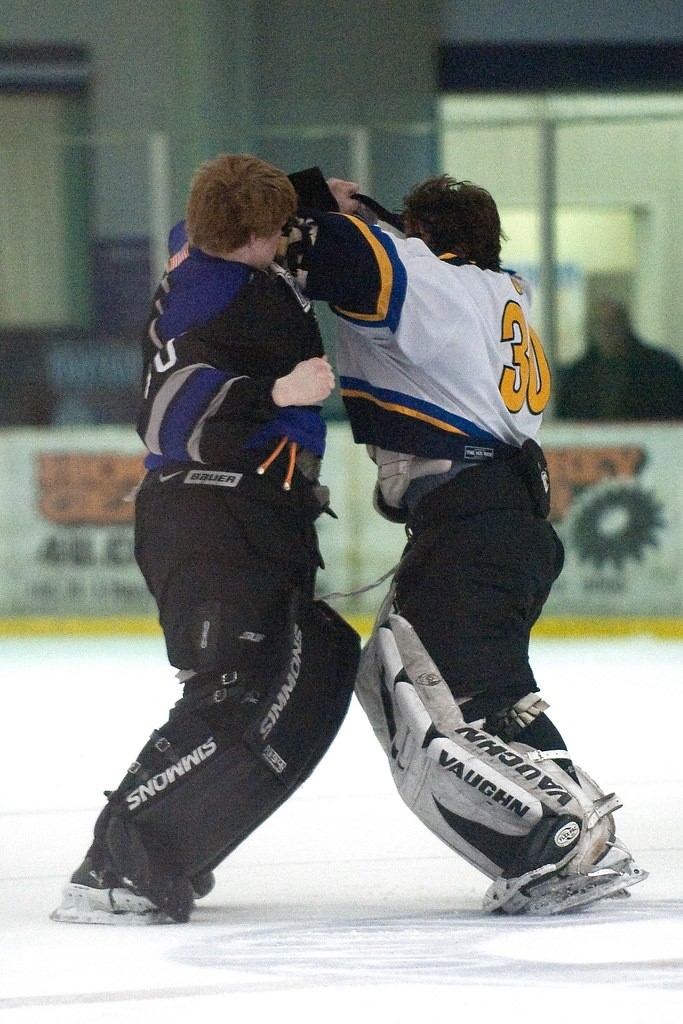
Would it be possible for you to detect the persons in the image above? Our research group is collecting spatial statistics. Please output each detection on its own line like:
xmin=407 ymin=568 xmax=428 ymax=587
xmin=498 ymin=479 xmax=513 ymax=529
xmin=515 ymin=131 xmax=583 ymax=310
xmin=272 ymin=172 xmax=649 ymax=917
xmin=50 ymin=155 xmax=362 ymax=925
xmin=557 ymin=293 xmax=683 ymax=421
